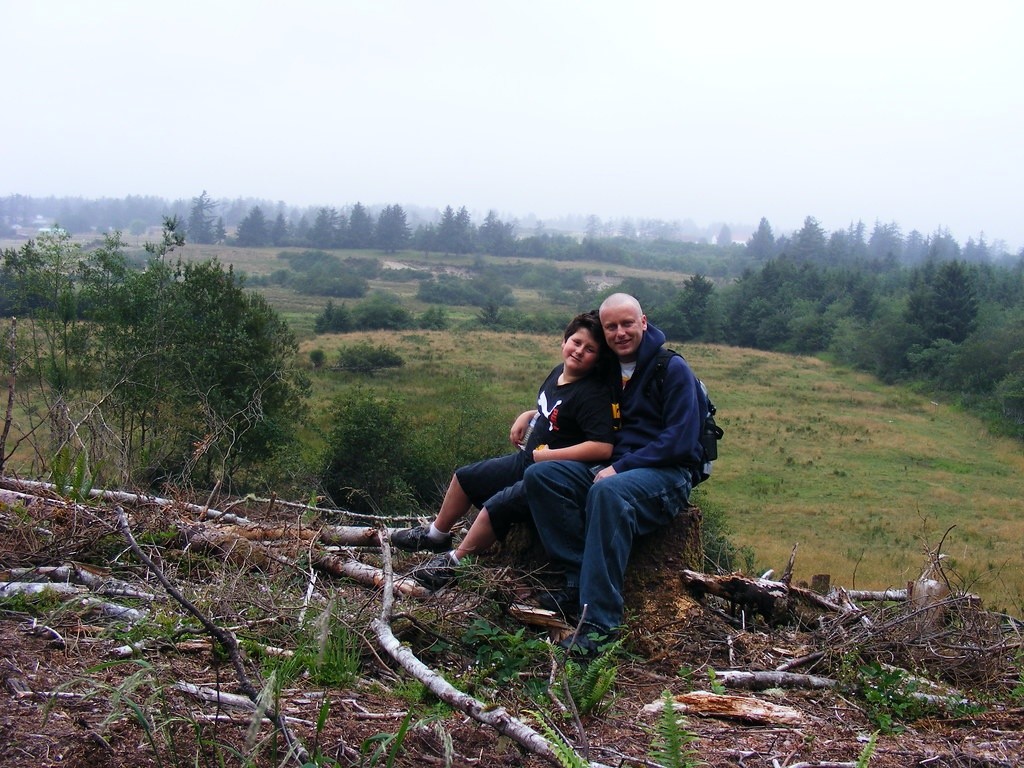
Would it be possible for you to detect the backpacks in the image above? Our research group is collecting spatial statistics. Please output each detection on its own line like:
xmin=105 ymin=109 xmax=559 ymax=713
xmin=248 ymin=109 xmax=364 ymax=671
xmin=655 ymin=349 xmax=724 ymax=487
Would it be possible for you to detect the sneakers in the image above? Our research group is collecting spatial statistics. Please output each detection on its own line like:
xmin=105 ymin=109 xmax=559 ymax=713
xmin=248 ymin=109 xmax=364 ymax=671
xmin=391 ymin=525 xmax=456 ymax=553
xmin=541 ymin=587 xmax=581 ymax=617
xmin=414 ymin=554 xmax=459 ymax=590
xmin=558 ymin=624 xmax=615 ymax=658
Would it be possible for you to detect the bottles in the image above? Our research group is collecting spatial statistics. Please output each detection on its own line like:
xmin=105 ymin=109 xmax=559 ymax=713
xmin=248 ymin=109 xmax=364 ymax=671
xmin=518 ymin=411 xmax=542 ymax=451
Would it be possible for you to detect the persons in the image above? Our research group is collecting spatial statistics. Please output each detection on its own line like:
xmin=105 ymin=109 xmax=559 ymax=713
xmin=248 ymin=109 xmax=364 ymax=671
xmin=509 ymin=293 xmax=719 ymax=661
xmin=388 ymin=310 xmax=613 ymax=591
